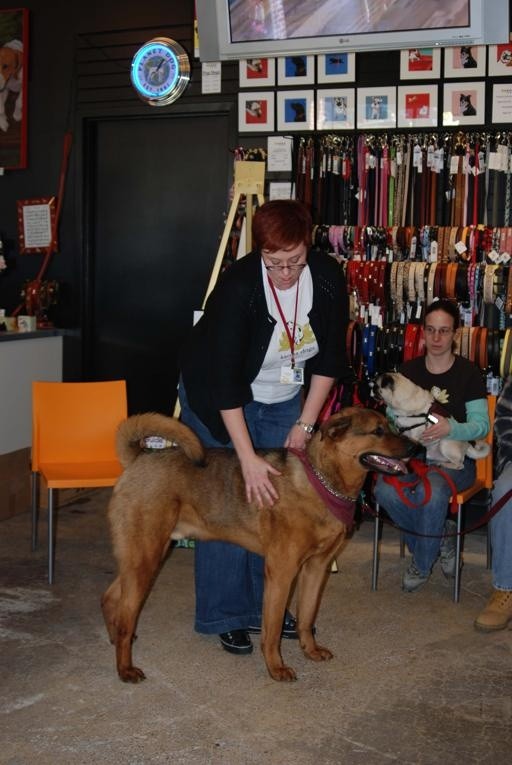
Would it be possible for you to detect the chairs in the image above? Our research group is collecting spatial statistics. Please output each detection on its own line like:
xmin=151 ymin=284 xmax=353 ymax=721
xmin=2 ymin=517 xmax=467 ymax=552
xmin=372 ymin=395 xmax=496 ymax=604
xmin=31 ymin=380 xmax=128 ymax=585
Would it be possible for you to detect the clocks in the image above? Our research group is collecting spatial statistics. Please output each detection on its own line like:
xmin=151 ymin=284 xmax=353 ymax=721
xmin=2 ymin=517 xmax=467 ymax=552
xmin=131 ymin=37 xmax=190 ymax=106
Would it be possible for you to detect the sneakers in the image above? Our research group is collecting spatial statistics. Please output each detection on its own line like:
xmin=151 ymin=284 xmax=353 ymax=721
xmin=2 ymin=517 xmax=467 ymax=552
xmin=219 ymin=629 xmax=254 ymax=654
xmin=440 ymin=519 xmax=464 ymax=578
xmin=401 ymin=555 xmax=431 ymax=592
xmin=247 ymin=615 xmax=317 ymax=639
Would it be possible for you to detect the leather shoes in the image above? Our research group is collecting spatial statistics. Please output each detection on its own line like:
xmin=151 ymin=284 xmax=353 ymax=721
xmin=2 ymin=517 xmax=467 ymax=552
xmin=472 ymin=589 xmax=512 ymax=631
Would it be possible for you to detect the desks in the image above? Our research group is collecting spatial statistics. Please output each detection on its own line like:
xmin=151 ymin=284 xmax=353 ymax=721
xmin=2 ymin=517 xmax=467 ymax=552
xmin=0 ymin=326 xmax=66 ymax=522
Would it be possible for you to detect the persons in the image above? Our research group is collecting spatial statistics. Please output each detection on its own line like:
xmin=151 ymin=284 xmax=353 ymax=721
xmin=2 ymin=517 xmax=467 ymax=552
xmin=178 ymin=198 xmax=351 ymax=657
xmin=472 ymin=361 xmax=512 ymax=631
xmin=372 ymin=298 xmax=491 ymax=590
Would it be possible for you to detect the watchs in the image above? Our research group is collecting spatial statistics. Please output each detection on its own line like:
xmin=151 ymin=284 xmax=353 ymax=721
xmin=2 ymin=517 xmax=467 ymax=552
xmin=293 ymin=421 xmax=317 ymax=436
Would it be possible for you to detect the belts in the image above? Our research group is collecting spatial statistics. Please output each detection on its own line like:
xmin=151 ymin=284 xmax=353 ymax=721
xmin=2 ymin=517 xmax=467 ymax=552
xmin=345 ymin=322 xmax=512 ymax=380
xmin=311 ymin=224 xmax=512 ymax=263
xmin=295 ymin=132 xmax=512 ymax=224
xmin=344 ymin=261 xmax=512 ymax=322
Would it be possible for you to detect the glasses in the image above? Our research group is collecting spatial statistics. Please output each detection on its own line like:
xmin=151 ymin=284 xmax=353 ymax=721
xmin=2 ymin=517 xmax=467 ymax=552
xmin=262 ymin=255 xmax=308 ymax=271
xmin=424 ymin=326 xmax=455 ymax=335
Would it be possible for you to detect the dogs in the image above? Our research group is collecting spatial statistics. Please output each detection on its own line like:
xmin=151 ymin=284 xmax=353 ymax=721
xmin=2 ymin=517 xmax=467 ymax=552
xmin=94 ymin=403 xmax=430 ymax=692
xmin=368 ymin=363 xmax=496 ymax=476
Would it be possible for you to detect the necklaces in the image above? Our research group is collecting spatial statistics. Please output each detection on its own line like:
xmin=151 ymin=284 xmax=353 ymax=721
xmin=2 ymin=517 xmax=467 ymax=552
xmin=426 ymin=356 xmax=453 ymax=373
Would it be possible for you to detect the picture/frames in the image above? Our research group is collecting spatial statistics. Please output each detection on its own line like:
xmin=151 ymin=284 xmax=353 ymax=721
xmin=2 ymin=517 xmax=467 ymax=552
xmin=192 ymin=0 xmax=201 ymax=61
xmin=0 ymin=8 xmax=28 ymax=172
xmin=235 ymin=36 xmax=508 ymax=137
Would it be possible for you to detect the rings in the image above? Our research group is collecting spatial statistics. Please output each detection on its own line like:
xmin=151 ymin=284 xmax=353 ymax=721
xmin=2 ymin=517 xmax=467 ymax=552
xmin=429 ymin=435 xmax=433 ymax=440
